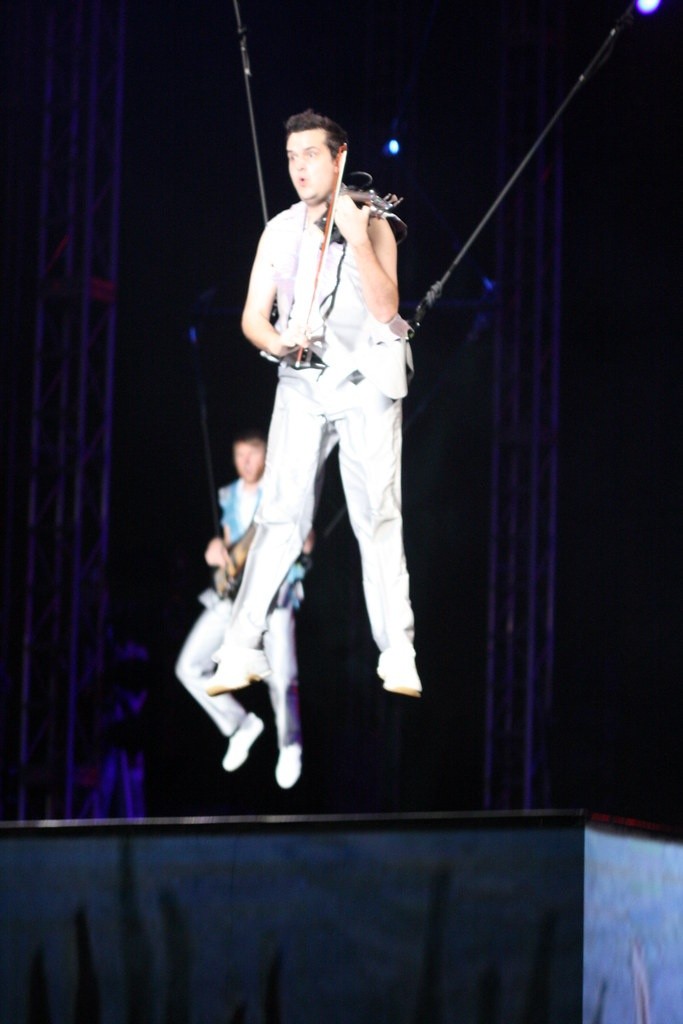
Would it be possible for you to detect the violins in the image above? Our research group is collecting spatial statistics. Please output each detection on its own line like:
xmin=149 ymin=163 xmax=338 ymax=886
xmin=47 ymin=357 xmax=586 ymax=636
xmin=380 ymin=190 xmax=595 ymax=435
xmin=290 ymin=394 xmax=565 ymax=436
xmin=318 ymin=185 xmax=405 ymax=245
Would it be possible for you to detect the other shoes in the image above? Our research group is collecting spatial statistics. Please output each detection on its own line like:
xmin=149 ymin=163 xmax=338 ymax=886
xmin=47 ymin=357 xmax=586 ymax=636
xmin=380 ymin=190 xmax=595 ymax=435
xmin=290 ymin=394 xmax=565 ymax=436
xmin=276 ymin=742 xmax=302 ymax=789
xmin=222 ymin=712 xmax=264 ymax=772
xmin=206 ymin=649 xmax=273 ymax=697
xmin=377 ymin=657 xmax=422 ymax=696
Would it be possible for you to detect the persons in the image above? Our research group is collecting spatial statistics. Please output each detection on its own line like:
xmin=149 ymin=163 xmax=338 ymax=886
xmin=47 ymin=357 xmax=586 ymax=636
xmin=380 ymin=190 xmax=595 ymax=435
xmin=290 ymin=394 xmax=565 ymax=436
xmin=170 ymin=435 xmax=312 ymax=790
xmin=207 ymin=112 xmax=424 ymax=695
